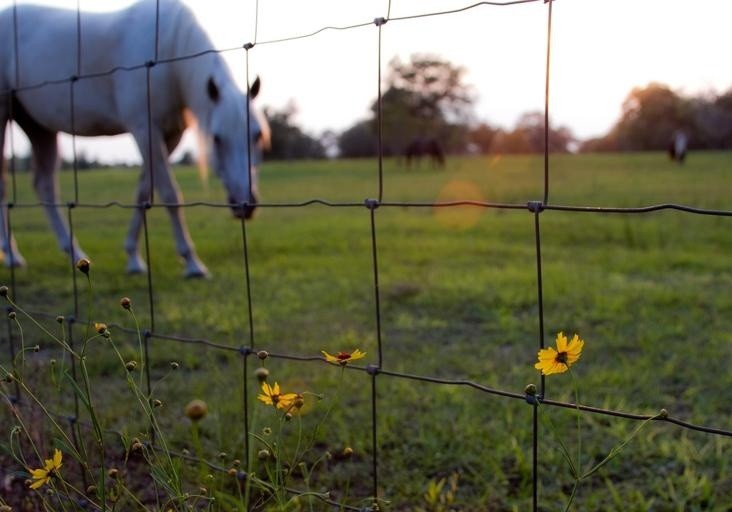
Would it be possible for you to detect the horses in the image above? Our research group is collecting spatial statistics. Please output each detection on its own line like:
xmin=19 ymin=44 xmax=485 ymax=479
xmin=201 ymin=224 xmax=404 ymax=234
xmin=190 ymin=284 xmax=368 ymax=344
xmin=0 ymin=0 xmax=272 ymax=283
xmin=405 ymin=136 xmax=448 ymax=170
